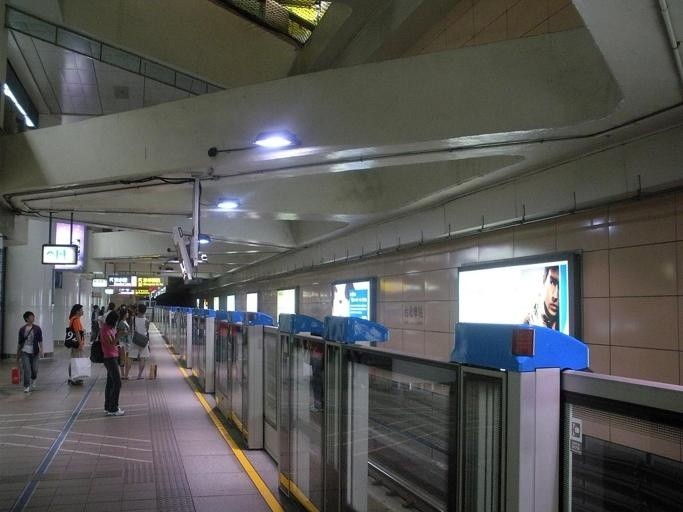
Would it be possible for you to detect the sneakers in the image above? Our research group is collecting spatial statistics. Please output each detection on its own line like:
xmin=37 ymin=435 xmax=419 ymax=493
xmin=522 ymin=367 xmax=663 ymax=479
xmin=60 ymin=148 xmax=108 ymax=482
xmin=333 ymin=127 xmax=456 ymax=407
xmin=32 ymin=380 xmax=36 ymax=387
xmin=24 ymin=387 xmax=29 ymax=392
xmin=104 ymin=408 xmax=125 ymax=416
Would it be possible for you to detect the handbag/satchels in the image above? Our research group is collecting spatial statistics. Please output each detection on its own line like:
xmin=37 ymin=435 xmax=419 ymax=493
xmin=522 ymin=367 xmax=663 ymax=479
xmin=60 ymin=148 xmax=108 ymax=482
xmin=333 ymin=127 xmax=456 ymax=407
xmin=65 ymin=327 xmax=83 ymax=348
xmin=11 ymin=367 xmax=21 ymax=384
xmin=132 ymin=331 xmax=149 ymax=347
xmin=91 ymin=342 xmax=105 ymax=363
xmin=70 ymin=358 xmax=91 ymax=382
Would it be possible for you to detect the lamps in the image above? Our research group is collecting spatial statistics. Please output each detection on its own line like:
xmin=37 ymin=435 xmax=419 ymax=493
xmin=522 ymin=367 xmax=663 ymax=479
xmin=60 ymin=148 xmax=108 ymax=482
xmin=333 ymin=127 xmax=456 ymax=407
xmin=248 ymin=130 xmax=303 ymax=151
xmin=213 ymin=197 xmax=240 ymax=210
xmin=197 ymin=234 xmax=211 ymax=245
xmin=166 ymin=256 xmax=179 ymax=264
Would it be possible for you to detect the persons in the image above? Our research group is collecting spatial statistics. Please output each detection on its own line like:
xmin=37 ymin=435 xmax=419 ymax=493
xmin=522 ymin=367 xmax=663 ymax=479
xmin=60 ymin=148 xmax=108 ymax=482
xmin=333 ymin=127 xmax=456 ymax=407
xmin=15 ymin=310 xmax=44 ymax=394
xmin=89 ymin=310 xmax=127 ymax=416
xmin=516 ymin=268 xmax=559 ymax=332
xmin=306 ymin=331 xmax=329 ymax=414
xmin=64 ymin=301 xmax=149 ymax=385
xmin=173 ymin=308 xmax=183 ymax=330
